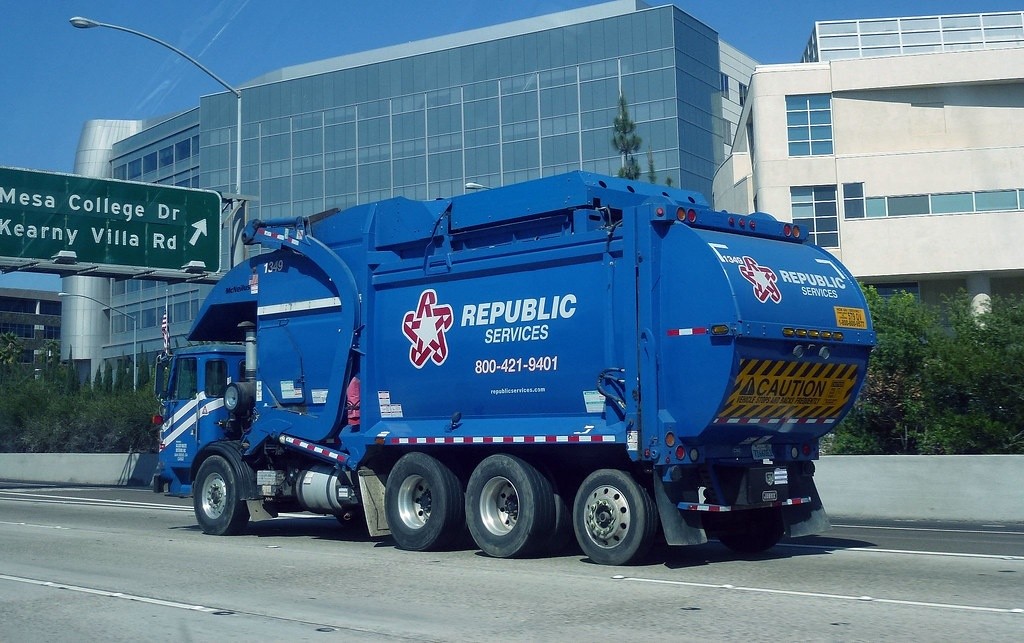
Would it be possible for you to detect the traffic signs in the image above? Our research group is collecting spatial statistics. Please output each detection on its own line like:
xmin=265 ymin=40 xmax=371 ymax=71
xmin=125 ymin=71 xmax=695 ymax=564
xmin=0 ymin=165 xmax=223 ymax=281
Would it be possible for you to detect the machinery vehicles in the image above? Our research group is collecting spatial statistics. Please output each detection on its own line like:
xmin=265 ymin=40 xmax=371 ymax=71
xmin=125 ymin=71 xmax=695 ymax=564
xmin=150 ymin=170 xmax=877 ymax=566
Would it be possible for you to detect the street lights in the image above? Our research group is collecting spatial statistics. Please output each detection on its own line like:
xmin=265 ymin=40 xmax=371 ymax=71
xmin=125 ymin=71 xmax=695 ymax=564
xmin=58 ymin=292 xmax=137 ymax=392
xmin=70 ymin=17 xmax=241 ymax=192
